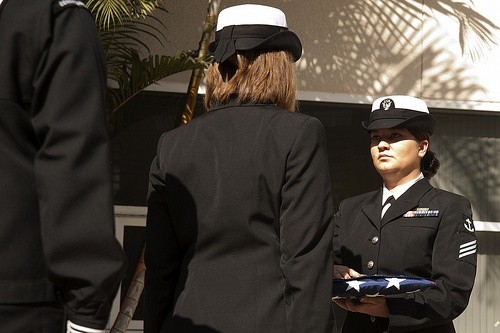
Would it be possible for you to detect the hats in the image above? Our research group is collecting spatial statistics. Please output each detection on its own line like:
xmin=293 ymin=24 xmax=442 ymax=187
xmin=208 ymin=4 xmax=302 ymax=82
xmin=362 ymin=95 xmax=434 ymax=136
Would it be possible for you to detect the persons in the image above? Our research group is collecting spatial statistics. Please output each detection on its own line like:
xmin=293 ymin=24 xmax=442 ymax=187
xmin=0 ymin=1 xmax=129 ymax=333
xmin=330 ymin=93 xmax=480 ymax=332
xmin=142 ymin=2 xmax=337 ymax=332
